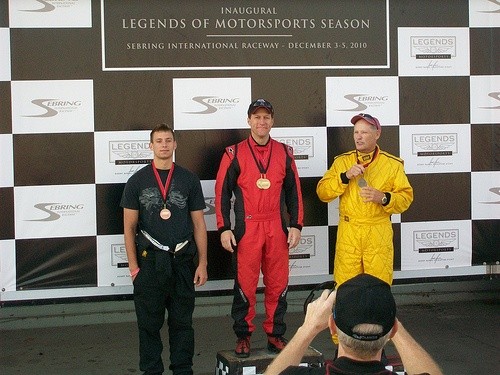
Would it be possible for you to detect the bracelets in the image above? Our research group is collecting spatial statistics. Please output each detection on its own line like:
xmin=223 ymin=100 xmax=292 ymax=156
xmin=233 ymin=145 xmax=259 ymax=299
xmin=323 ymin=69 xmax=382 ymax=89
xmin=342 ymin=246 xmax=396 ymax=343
xmin=129 ymin=267 xmax=140 ymax=277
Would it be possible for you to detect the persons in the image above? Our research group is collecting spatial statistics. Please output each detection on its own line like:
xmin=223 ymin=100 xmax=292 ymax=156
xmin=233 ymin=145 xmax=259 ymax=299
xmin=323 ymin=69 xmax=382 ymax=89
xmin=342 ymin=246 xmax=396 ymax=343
xmin=315 ymin=113 xmax=414 ymax=366
xmin=215 ymin=99 xmax=304 ymax=358
xmin=261 ymin=273 xmax=443 ymax=375
xmin=120 ymin=122 xmax=208 ymax=375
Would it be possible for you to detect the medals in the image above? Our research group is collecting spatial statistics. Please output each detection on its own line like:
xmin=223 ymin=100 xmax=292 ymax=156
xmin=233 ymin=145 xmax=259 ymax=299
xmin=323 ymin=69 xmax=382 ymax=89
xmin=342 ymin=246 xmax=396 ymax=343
xmin=255 ymin=174 xmax=271 ymax=189
xmin=359 ymin=178 xmax=367 ymax=186
xmin=160 ymin=209 xmax=172 ymax=220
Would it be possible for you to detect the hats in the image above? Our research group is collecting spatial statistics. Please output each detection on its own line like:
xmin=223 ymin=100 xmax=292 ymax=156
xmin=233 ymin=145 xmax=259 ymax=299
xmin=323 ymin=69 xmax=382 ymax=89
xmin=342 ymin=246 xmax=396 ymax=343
xmin=248 ymin=99 xmax=274 ymax=114
xmin=333 ymin=273 xmax=396 ymax=341
xmin=351 ymin=114 xmax=381 ymax=130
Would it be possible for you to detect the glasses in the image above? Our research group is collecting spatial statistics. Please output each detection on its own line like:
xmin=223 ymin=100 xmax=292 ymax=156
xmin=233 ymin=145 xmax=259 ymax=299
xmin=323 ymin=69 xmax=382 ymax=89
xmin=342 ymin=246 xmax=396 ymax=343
xmin=359 ymin=113 xmax=378 ymax=130
xmin=304 ymin=281 xmax=337 ymax=315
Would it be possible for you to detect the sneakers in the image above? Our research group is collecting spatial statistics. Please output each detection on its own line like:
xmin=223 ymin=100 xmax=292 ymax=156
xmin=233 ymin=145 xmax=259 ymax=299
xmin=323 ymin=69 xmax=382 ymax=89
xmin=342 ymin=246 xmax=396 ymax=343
xmin=235 ymin=336 xmax=251 ymax=358
xmin=267 ymin=336 xmax=289 ymax=354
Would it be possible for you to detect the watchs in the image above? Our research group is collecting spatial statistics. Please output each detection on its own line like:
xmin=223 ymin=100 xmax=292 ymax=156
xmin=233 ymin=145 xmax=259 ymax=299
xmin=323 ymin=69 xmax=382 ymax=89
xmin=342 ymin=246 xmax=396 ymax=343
xmin=381 ymin=194 xmax=387 ymax=205
